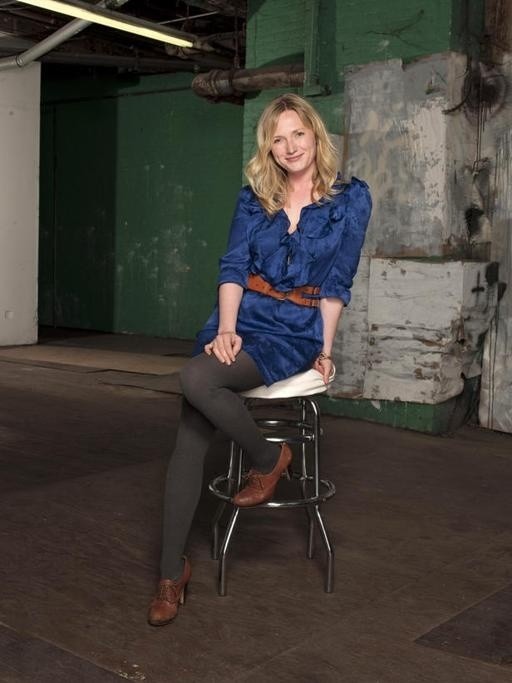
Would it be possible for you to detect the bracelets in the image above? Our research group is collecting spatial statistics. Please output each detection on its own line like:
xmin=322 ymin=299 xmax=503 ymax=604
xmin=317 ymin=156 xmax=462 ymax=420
xmin=321 ymin=353 xmax=331 ymax=360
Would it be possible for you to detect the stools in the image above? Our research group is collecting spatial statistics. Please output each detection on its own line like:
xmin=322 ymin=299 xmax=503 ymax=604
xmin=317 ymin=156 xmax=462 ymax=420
xmin=210 ymin=360 xmax=341 ymax=594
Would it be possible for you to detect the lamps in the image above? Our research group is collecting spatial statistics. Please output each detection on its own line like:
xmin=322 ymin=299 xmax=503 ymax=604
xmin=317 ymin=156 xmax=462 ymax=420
xmin=19 ymin=0 xmax=199 ymax=48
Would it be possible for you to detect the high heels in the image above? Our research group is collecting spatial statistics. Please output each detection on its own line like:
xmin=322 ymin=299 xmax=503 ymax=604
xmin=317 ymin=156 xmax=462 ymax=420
xmin=234 ymin=443 xmax=293 ymax=507
xmin=148 ymin=555 xmax=191 ymax=625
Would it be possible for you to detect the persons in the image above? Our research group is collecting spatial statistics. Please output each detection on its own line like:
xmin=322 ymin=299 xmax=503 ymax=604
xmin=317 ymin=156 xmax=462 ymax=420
xmin=148 ymin=93 xmax=372 ymax=628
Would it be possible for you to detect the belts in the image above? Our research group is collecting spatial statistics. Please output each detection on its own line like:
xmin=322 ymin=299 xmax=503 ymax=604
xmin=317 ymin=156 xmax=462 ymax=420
xmin=247 ymin=274 xmax=321 ymax=307
